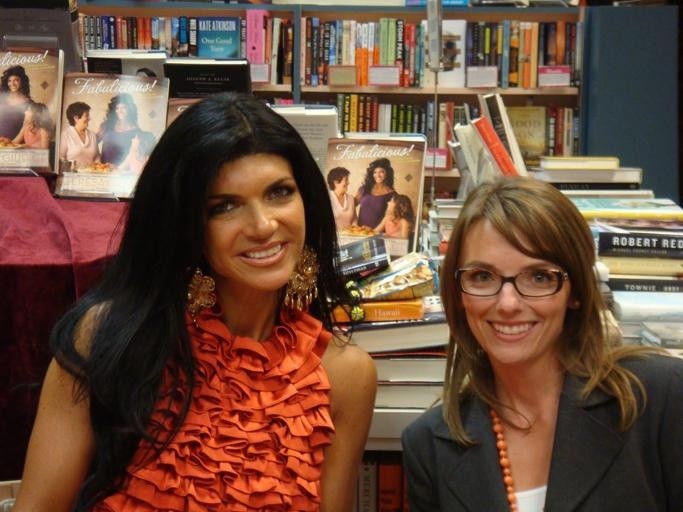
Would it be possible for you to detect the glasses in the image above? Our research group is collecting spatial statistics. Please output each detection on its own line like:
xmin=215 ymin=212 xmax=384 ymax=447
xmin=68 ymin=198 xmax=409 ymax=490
xmin=450 ymin=259 xmax=574 ymax=298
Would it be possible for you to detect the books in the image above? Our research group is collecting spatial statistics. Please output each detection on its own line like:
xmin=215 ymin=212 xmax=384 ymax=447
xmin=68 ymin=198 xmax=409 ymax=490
xmin=76 ymin=9 xmax=292 ymax=87
xmin=302 ymin=14 xmax=583 ymax=90
xmin=320 ymin=131 xmax=682 ymax=443
xmin=335 ymin=93 xmax=580 ymax=171
xmin=354 ymin=463 xmax=408 ymax=512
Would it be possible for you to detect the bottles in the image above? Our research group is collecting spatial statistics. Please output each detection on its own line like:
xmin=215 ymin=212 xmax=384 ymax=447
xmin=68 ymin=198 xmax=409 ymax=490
xmin=593 ymin=261 xmax=614 ymax=316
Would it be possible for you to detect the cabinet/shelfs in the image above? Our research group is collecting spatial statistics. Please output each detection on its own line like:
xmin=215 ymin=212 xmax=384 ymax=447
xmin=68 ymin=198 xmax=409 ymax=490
xmin=69 ymin=1 xmax=585 ymax=240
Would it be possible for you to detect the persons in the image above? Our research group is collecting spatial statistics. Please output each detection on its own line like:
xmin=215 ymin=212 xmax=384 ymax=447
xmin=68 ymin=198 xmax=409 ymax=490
xmin=0 ymin=64 xmax=36 ymax=147
xmin=96 ymin=93 xmax=137 ymax=166
xmin=5 ymin=91 xmax=379 ymax=512
xmin=59 ymin=101 xmax=98 ymax=168
xmin=10 ymin=103 xmax=53 ymax=150
xmin=400 ymin=176 xmax=683 ymax=512
xmin=116 ymin=130 xmax=157 ymax=171
